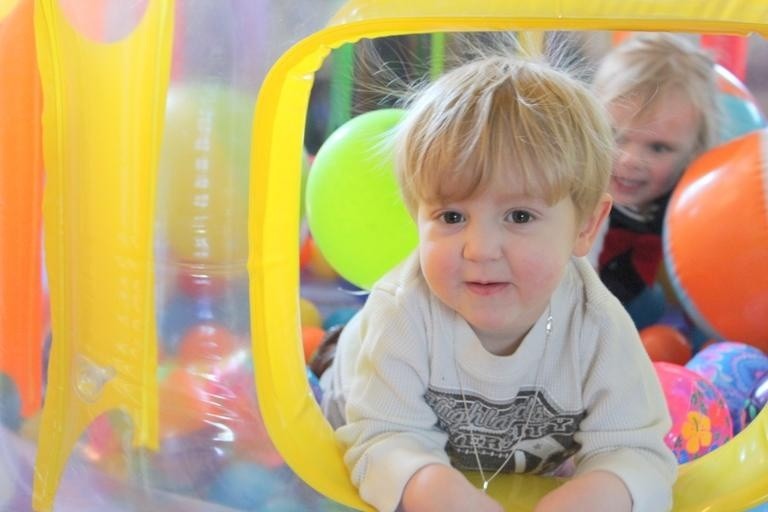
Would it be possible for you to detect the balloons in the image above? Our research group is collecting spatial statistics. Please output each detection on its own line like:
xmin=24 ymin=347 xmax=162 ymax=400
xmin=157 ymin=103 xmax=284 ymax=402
xmin=304 ymin=106 xmax=421 ymax=292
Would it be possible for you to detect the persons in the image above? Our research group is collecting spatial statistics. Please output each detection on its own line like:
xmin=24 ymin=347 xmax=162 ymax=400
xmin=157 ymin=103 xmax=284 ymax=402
xmin=589 ymin=32 xmax=723 ymax=306
xmin=319 ymin=53 xmax=682 ymax=512
